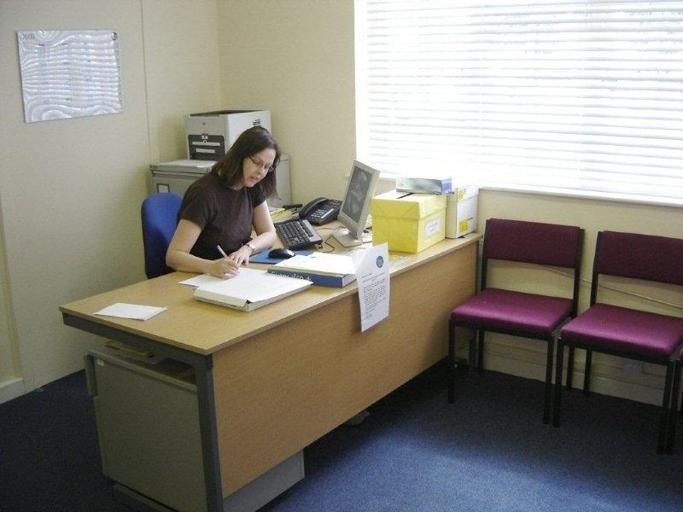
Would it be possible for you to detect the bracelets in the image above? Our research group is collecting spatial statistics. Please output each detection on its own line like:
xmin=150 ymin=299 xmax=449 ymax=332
xmin=244 ymin=242 xmax=257 ymax=255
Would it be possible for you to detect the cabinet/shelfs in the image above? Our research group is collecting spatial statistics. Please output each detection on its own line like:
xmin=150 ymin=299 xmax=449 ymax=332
xmin=151 ymin=155 xmax=291 ymax=221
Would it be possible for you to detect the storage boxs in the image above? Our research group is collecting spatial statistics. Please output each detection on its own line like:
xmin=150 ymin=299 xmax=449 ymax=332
xmin=445 ymin=185 xmax=479 ymax=239
xmin=372 ymin=191 xmax=445 ymax=254
xmin=186 ymin=109 xmax=271 ymax=162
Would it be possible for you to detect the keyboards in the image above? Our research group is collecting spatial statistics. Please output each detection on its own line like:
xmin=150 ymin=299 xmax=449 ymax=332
xmin=274 ymin=219 xmax=323 ymax=251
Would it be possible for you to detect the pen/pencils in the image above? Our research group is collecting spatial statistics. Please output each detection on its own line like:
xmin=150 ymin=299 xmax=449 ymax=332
xmin=216 ymin=244 xmax=229 ymax=259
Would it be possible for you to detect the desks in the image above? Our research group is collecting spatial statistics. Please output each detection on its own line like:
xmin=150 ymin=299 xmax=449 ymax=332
xmin=59 ymin=206 xmax=483 ymax=511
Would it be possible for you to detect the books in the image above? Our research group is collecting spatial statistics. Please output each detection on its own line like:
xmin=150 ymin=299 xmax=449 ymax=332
xmin=195 ymin=272 xmax=315 ymax=313
xmin=267 ymin=251 xmax=356 ymax=288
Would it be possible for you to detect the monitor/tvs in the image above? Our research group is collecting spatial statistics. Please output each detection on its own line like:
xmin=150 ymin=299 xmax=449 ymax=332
xmin=331 ymin=159 xmax=381 ymax=248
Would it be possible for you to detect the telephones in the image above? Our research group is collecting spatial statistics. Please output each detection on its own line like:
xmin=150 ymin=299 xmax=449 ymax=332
xmin=298 ymin=197 xmax=343 ymax=225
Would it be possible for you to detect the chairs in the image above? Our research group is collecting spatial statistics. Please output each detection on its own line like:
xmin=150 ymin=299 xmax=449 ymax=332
xmin=553 ymin=231 xmax=682 ymax=455
xmin=449 ymin=219 xmax=581 ymax=423
xmin=665 ymin=348 xmax=683 ymax=455
xmin=140 ymin=192 xmax=190 ymax=280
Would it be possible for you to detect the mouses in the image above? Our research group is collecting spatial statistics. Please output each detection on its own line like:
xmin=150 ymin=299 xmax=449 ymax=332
xmin=268 ymin=248 xmax=295 ymax=259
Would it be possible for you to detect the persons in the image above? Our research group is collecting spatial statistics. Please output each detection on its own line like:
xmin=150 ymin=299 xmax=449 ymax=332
xmin=164 ymin=126 xmax=283 ymax=280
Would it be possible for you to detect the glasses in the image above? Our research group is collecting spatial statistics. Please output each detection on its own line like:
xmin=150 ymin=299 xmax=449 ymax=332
xmin=248 ymin=156 xmax=274 ymax=173
